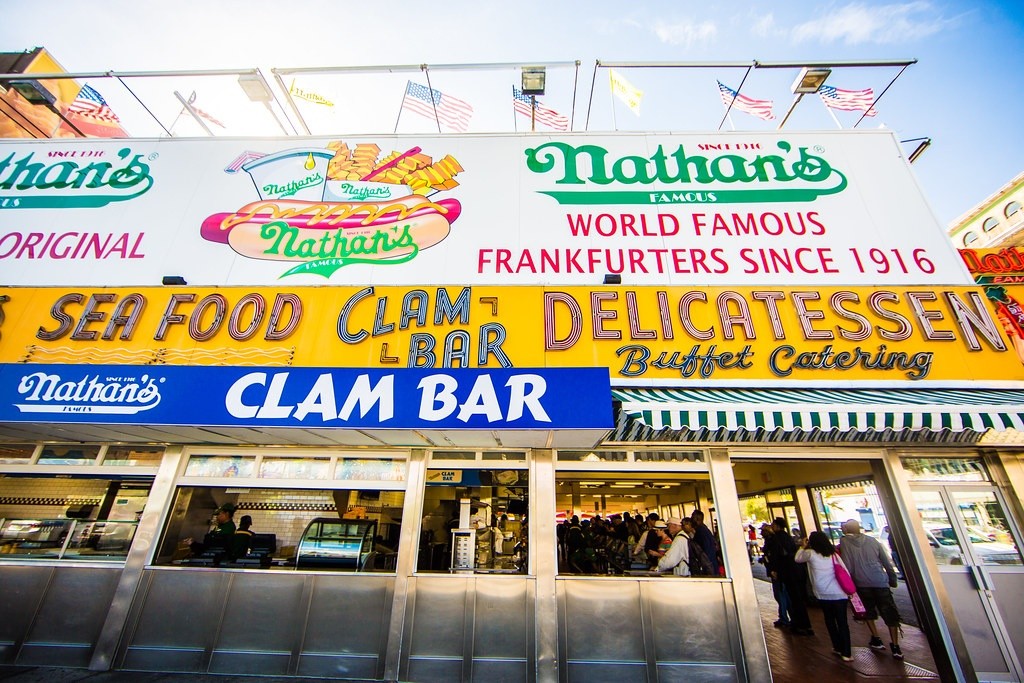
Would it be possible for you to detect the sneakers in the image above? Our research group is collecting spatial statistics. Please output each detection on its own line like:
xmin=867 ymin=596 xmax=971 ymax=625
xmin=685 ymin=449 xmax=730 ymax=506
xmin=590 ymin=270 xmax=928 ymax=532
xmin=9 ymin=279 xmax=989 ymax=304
xmin=890 ymin=643 xmax=904 ymax=657
xmin=870 ymin=636 xmax=886 ymax=650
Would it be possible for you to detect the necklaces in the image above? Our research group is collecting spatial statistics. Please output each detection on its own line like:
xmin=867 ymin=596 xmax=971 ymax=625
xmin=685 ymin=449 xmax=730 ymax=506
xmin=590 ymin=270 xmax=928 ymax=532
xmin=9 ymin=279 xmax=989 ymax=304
xmin=758 ymin=516 xmax=818 ymax=635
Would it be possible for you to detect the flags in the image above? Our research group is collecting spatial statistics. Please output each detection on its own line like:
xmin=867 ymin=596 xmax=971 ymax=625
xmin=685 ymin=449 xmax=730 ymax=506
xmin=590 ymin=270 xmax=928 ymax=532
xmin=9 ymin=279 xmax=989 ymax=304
xmin=403 ymin=81 xmax=473 ymax=132
xmin=818 ymin=86 xmax=875 ymax=114
xmin=290 ymin=78 xmax=333 ymax=107
xmin=513 ymin=85 xmax=568 ymax=130
xmin=716 ymin=80 xmax=775 ymax=121
xmin=609 ymin=70 xmax=642 ymax=111
xmin=68 ymin=84 xmax=119 ymax=122
xmin=189 ymin=89 xmax=226 ymax=129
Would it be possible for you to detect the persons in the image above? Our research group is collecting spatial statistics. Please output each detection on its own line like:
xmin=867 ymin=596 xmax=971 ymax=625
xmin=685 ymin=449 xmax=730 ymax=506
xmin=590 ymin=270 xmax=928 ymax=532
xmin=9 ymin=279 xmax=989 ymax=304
xmin=743 ymin=525 xmax=760 ymax=556
xmin=651 ymin=516 xmax=690 ymax=577
xmin=837 ymin=519 xmax=903 ymax=659
xmin=183 ymin=503 xmax=256 ymax=556
xmin=681 ymin=510 xmax=720 ymax=574
xmin=447 ymin=511 xmax=459 ymax=548
xmin=491 ymin=514 xmax=507 ymax=526
xmin=795 ymin=532 xmax=854 ymax=662
xmin=885 ymin=526 xmax=905 ymax=580
xmin=557 ymin=512 xmax=673 ymax=574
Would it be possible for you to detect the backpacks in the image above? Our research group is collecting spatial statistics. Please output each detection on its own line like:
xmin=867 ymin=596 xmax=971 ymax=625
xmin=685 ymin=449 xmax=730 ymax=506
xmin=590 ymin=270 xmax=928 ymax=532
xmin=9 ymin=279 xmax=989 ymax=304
xmin=677 ymin=534 xmax=714 ymax=575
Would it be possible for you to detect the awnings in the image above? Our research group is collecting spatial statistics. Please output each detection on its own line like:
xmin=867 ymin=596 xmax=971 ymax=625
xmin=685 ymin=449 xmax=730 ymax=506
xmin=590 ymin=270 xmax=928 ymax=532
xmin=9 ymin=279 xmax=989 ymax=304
xmin=611 ymin=387 xmax=1024 ymax=442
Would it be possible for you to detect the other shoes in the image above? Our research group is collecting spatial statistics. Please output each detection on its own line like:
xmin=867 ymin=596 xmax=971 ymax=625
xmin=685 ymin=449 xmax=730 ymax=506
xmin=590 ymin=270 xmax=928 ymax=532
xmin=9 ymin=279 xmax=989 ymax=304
xmin=773 ymin=618 xmax=789 ymax=625
xmin=842 ymin=655 xmax=854 ymax=661
xmin=832 ymin=648 xmax=842 ymax=655
xmin=796 ymin=628 xmax=815 ymax=636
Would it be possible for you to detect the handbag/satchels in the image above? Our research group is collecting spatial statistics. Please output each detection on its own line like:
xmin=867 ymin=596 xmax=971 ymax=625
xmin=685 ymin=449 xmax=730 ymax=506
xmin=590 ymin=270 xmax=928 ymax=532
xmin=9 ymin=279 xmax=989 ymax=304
xmin=848 ymin=593 xmax=866 ymax=618
xmin=832 ymin=553 xmax=856 ymax=594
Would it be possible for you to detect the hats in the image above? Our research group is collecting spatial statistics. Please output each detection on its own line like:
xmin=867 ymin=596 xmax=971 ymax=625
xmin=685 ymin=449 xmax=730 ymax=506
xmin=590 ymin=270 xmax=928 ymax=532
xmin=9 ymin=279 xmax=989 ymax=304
xmin=765 ymin=526 xmax=773 ymax=533
xmin=212 ymin=502 xmax=235 ymax=515
xmin=652 ymin=520 xmax=668 ymax=530
xmin=758 ymin=523 xmax=768 ymax=530
xmin=664 ymin=517 xmax=682 ymax=526
xmin=570 ymin=515 xmax=579 ymax=524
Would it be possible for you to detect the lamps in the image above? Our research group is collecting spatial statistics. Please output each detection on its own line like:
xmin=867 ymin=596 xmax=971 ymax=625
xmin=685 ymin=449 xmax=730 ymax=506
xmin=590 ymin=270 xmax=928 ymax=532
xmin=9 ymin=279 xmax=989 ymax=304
xmin=237 ymin=74 xmax=290 ymax=136
xmin=778 ymin=67 xmax=833 ymax=130
xmin=523 ymin=66 xmax=546 ymax=130
xmin=8 ymin=80 xmax=86 ymax=138
